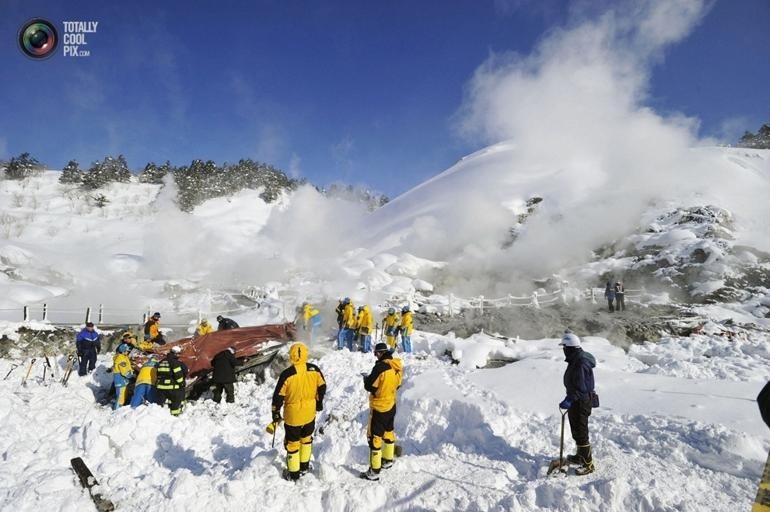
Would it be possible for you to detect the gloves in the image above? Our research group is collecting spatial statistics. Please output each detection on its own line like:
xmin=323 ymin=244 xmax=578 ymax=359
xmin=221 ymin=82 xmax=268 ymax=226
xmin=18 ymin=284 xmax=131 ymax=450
xmin=560 ymin=397 xmax=572 ymax=408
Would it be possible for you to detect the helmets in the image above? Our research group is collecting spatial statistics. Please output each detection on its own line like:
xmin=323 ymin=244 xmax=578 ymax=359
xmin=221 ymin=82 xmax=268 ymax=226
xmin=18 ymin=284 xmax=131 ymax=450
xmin=359 ymin=305 xmax=364 ymax=309
xmin=339 ymin=297 xmax=345 ymax=304
xmin=201 ymin=319 xmax=207 ymax=323
xmin=119 ymin=344 xmax=131 ymax=352
xmin=345 ymin=296 xmax=352 ymax=305
xmin=154 ymin=313 xmax=160 ymax=317
xmin=172 ymin=345 xmax=181 ymax=354
xmin=403 ymin=306 xmax=409 ymax=312
xmin=389 ymin=308 xmax=395 ymax=314
xmin=217 ymin=316 xmax=223 ymax=320
xmin=559 ymin=334 xmax=581 ymax=347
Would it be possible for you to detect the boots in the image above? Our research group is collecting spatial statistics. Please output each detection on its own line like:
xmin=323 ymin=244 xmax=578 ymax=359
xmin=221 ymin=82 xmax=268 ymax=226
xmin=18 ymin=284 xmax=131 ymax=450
xmin=575 ymin=444 xmax=595 ymax=475
xmin=567 ymin=453 xmax=584 ymax=465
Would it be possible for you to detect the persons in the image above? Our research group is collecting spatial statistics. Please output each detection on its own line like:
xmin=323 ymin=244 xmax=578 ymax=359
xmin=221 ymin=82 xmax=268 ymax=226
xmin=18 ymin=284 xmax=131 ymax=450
xmin=104 ymin=310 xmax=241 ymax=417
xmin=756 ymin=381 xmax=770 ymax=428
xmin=604 ymin=281 xmax=617 ymax=311
xmin=360 ymin=344 xmax=404 ymax=481
xmin=75 ymin=322 xmax=101 ymax=376
xmin=335 ymin=296 xmax=412 ymax=355
xmin=558 ymin=334 xmax=596 ymax=474
xmin=615 ymin=282 xmax=626 ymax=312
xmin=301 ymin=301 xmax=321 ymax=342
xmin=271 ymin=341 xmax=326 ymax=483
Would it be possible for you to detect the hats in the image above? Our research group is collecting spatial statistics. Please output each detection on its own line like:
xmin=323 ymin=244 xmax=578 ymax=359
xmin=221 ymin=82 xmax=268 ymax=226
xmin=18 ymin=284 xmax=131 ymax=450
xmin=375 ymin=343 xmax=388 ymax=351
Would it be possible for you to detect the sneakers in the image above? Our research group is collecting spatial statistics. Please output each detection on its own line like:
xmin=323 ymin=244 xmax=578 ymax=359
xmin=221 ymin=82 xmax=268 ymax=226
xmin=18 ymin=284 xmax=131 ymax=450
xmin=360 ymin=466 xmax=379 ymax=480
xmin=283 ymin=468 xmax=292 ymax=480
xmin=381 ymin=459 xmax=392 ymax=468
